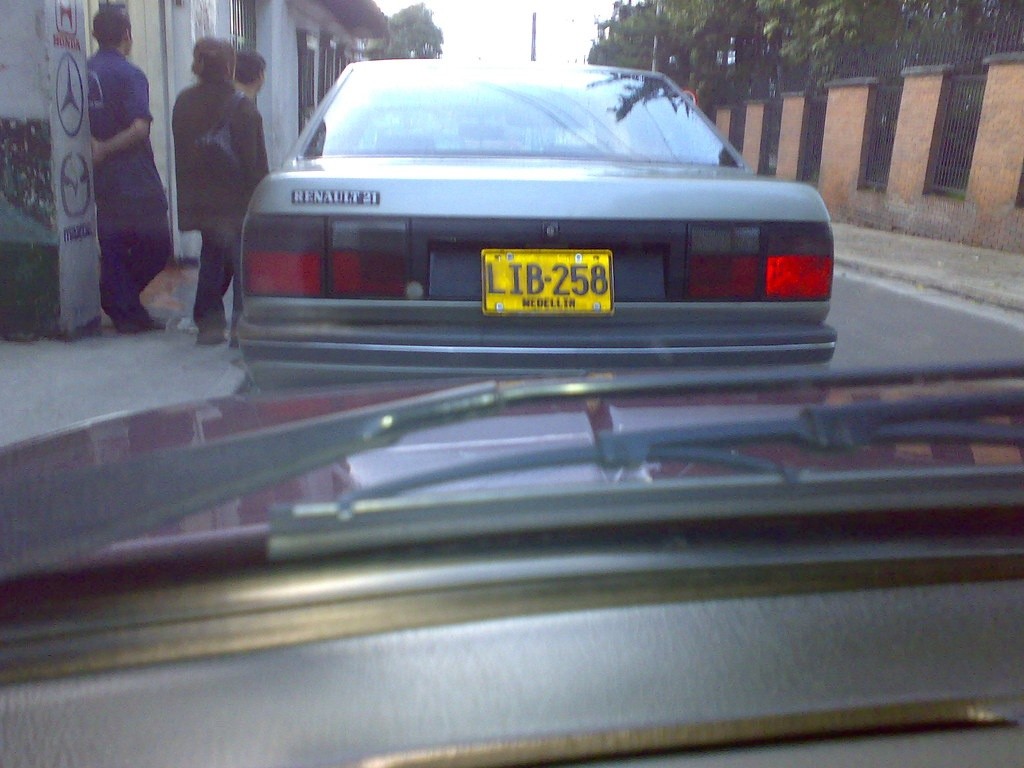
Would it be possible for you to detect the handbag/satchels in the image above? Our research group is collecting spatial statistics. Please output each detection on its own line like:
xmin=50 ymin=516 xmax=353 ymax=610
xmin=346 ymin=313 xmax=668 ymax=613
xmin=195 ymin=91 xmax=249 ymax=184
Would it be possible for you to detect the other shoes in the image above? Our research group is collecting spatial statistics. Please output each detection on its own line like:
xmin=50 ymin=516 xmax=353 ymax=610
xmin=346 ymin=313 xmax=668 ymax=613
xmin=197 ymin=323 xmax=229 ymax=344
xmin=117 ymin=318 xmax=166 ymax=334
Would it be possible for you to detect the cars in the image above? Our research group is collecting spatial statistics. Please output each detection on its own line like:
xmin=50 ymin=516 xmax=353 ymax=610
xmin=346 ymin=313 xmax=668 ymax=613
xmin=234 ymin=59 xmax=838 ymax=399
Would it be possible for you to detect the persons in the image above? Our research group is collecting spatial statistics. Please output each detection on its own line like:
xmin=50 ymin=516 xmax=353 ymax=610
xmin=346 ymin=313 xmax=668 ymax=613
xmin=172 ymin=35 xmax=270 ymax=349
xmin=86 ymin=3 xmax=174 ymax=333
